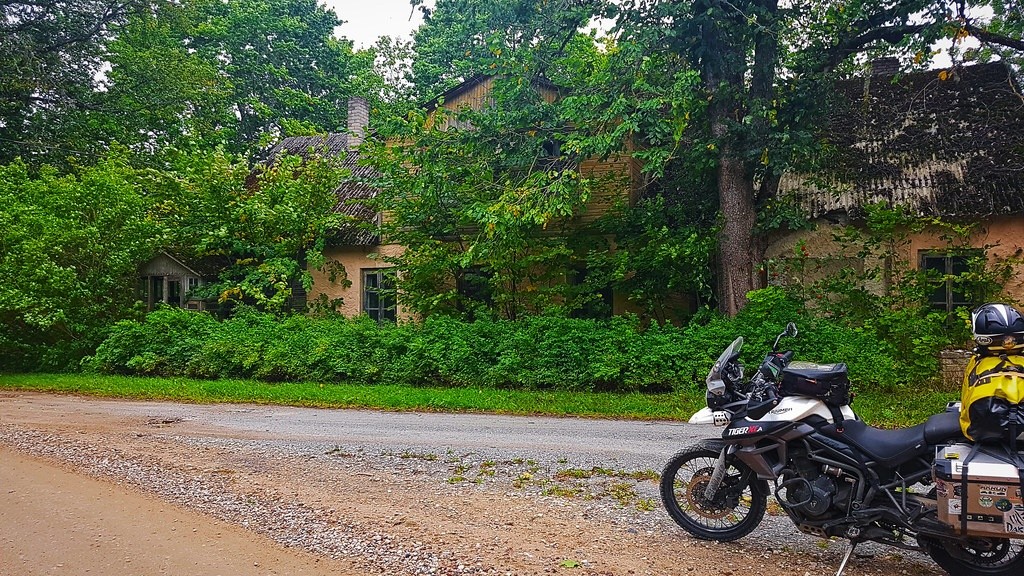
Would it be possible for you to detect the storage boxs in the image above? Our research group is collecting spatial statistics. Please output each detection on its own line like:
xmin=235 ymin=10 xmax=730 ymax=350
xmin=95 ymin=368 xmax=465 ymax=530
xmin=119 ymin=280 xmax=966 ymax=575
xmin=930 ymin=442 xmax=1024 ymax=540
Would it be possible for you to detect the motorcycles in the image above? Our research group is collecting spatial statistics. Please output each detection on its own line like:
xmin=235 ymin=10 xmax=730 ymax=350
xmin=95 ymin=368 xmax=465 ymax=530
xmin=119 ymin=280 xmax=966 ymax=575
xmin=662 ymin=322 xmax=1024 ymax=576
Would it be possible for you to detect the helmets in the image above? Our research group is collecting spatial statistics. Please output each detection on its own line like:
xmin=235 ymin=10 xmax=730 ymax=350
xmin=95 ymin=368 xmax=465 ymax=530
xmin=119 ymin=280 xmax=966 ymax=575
xmin=971 ymin=303 xmax=1024 ymax=348
